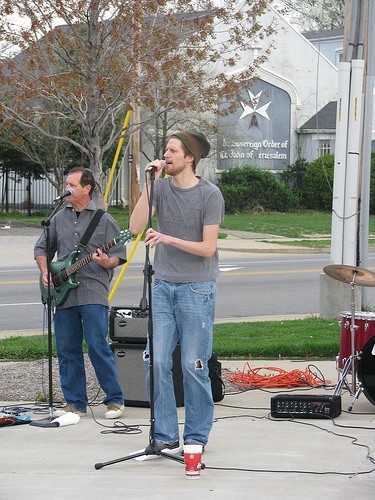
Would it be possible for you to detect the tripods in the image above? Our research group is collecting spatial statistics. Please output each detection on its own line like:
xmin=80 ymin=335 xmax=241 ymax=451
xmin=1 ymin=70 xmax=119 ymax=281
xmin=333 ymin=273 xmax=359 ymax=396
xmin=94 ymin=172 xmax=206 ymax=470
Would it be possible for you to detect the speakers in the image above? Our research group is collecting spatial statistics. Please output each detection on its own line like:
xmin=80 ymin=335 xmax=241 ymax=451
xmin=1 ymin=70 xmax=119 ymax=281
xmin=109 ymin=307 xmax=149 ymax=344
xmin=109 ymin=343 xmax=224 ymax=408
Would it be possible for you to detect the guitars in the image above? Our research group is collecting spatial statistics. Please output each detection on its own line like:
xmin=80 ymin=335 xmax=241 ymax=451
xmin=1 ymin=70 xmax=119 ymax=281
xmin=39 ymin=228 xmax=133 ymax=307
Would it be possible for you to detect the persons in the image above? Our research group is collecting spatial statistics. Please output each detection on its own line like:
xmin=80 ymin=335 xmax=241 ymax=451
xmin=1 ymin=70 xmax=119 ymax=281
xmin=129 ymin=129 xmax=225 ymax=460
xmin=34 ymin=167 xmax=128 ymax=418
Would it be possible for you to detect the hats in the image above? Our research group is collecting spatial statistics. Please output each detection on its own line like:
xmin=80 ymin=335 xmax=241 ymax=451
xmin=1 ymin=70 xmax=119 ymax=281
xmin=171 ymin=129 xmax=210 ymax=167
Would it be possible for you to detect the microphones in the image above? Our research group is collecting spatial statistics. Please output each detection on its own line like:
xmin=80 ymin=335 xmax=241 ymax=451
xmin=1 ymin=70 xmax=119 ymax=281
xmin=146 ymin=160 xmax=167 ymax=173
xmin=54 ymin=188 xmax=74 ymax=201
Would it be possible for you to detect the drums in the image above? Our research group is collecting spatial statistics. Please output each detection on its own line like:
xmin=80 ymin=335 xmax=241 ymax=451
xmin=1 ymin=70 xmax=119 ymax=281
xmin=358 ymin=334 xmax=375 ymax=406
xmin=336 ymin=310 xmax=375 ymax=374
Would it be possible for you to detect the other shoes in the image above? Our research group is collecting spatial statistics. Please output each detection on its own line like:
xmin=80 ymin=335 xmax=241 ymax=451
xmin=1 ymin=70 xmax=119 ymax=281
xmin=105 ymin=403 xmax=124 ymax=419
xmin=145 ymin=440 xmax=179 ymax=454
xmin=181 ymin=441 xmax=204 ymax=456
xmin=54 ymin=404 xmax=86 ymax=417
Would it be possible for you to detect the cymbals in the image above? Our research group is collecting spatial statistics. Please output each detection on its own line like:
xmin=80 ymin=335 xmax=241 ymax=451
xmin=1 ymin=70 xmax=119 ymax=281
xmin=323 ymin=265 xmax=375 ymax=287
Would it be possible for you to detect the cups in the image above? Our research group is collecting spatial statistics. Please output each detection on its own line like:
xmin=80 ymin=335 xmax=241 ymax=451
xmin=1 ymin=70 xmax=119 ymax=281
xmin=184 ymin=444 xmax=203 ymax=480
